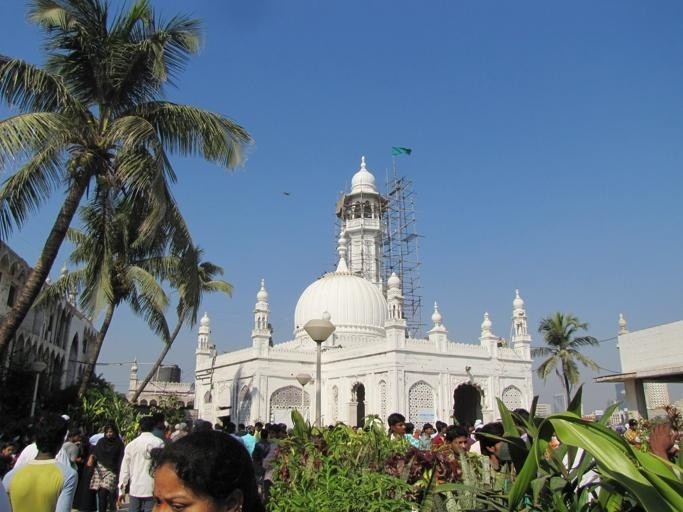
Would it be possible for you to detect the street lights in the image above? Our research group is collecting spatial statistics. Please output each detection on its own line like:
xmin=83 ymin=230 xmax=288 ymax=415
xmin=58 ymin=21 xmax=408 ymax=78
xmin=302 ymin=317 xmax=337 ymax=428
xmin=25 ymin=360 xmax=51 ymax=417
xmin=294 ymin=371 xmax=312 ymax=418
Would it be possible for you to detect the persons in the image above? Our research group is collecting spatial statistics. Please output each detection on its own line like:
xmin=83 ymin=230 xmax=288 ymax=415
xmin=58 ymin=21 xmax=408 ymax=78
xmin=0 ymin=412 xmax=290 ymax=511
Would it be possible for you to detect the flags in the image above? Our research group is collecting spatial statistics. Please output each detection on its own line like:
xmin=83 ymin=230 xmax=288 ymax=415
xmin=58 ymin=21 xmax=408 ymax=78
xmin=391 ymin=144 xmax=412 ymax=157
